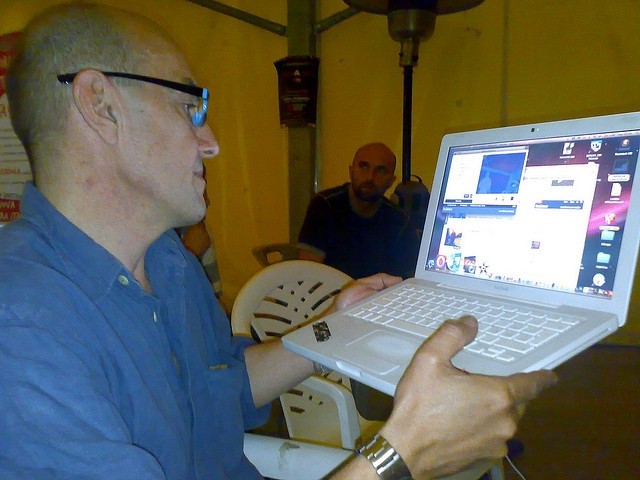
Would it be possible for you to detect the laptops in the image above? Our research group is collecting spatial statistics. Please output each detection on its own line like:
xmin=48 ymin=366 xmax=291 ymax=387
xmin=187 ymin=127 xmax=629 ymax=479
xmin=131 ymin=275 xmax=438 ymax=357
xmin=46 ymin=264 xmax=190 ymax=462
xmin=280 ymin=111 xmax=640 ymax=399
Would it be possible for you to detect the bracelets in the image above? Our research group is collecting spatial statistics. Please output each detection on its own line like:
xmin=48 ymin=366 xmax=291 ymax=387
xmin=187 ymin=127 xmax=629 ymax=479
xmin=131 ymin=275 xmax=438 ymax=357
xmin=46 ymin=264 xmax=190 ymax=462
xmin=356 ymin=432 xmax=414 ymax=479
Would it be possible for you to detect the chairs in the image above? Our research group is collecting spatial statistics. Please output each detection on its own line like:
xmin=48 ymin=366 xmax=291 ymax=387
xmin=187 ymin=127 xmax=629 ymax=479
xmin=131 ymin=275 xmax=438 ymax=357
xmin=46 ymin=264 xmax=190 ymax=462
xmin=230 ymin=260 xmax=507 ymax=480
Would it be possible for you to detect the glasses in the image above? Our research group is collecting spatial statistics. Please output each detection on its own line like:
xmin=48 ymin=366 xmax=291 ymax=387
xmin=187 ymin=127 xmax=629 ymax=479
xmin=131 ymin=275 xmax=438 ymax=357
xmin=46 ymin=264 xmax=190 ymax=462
xmin=58 ymin=71 xmax=209 ymax=128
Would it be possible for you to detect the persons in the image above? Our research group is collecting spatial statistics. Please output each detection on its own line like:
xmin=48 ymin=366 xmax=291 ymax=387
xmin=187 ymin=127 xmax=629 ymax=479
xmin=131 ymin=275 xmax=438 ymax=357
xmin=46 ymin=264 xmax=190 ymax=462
xmin=295 ymin=142 xmax=421 ymax=279
xmin=174 ymin=166 xmax=222 ymax=301
xmin=1 ymin=3 xmax=563 ymax=480
